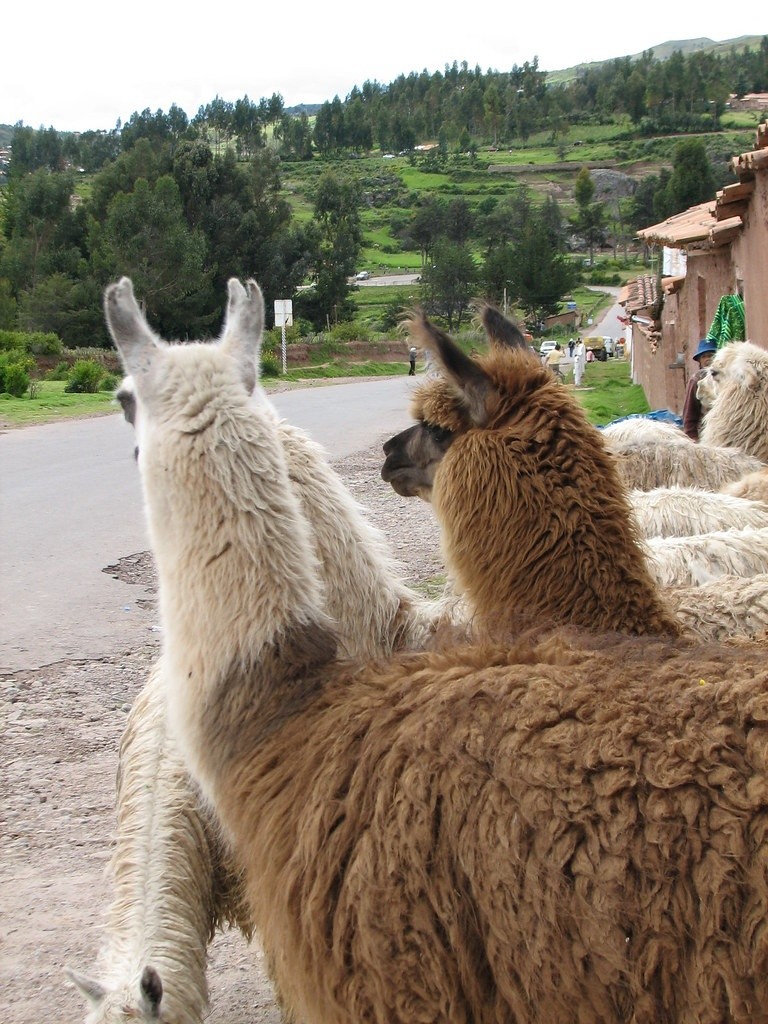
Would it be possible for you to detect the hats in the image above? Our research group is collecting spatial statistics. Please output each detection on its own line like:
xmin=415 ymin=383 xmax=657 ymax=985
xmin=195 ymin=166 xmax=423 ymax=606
xmin=693 ymin=339 xmax=718 ymax=361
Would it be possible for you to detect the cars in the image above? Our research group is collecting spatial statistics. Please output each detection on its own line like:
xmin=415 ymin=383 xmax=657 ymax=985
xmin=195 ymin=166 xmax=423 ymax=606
xmin=540 ymin=341 xmax=558 ymax=356
xmin=357 ymin=270 xmax=370 ymax=281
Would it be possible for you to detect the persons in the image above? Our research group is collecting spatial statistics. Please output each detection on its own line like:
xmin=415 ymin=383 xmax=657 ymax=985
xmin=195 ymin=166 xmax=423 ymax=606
xmin=406 ymin=335 xmax=627 ymax=386
xmin=681 ymin=338 xmax=719 ymax=440
